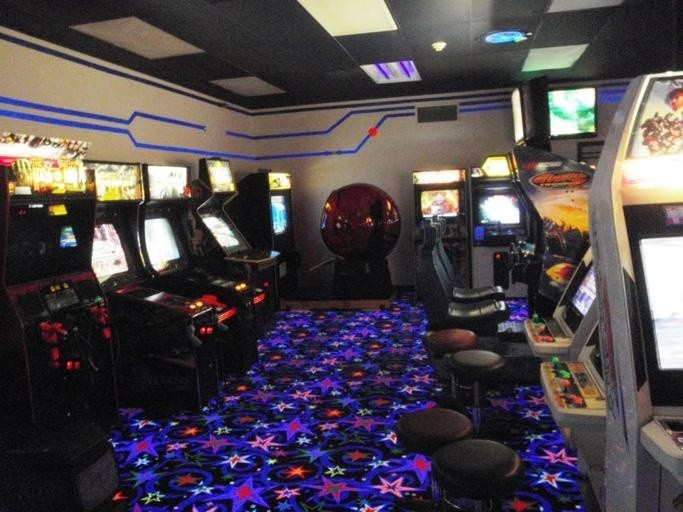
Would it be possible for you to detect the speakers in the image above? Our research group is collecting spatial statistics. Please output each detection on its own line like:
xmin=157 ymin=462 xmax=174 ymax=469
xmin=417 ymin=105 xmax=457 ymax=123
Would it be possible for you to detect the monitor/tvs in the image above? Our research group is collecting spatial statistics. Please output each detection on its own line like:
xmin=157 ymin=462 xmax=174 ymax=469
xmin=474 ymin=183 xmax=527 ymax=229
xmin=418 ymin=188 xmax=463 ymax=222
xmin=137 ymin=213 xmax=184 ymax=271
xmin=563 ymin=261 xmax=597 ymax=333
xmin=623 ymin=203 xmax=683 ymax=405
xmin=271 ymin=194 xmax=289 ymax=237
xmin=510 ymin=86 xmax=525 ymax=144
xmin=90 ymin=218 xmax=136 ymax=288
xmin=198 ymin=210 xmax=248 ymax=253
xmin=548 ymin=86 xmax=598 ymax=139
xmin=5 ymin=201 xmax=91 ymax=287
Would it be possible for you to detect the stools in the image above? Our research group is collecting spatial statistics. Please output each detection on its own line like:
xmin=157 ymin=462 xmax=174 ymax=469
xmin=394 ymin=403 xmax=473 ymax=509
xmin=432 ymin=435 xmax=524 ymax=512
xmin=412 ymin=326 xmax=473 ymax=407
xmin=444 ymin=343 xmax=515 ymax=438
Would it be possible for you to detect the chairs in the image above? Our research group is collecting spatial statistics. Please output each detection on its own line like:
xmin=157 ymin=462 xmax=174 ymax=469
xmin=423 ymin=212 xmax=505 ymax=302
xmin=412 ymin=220 xmax=507 ymax=339
